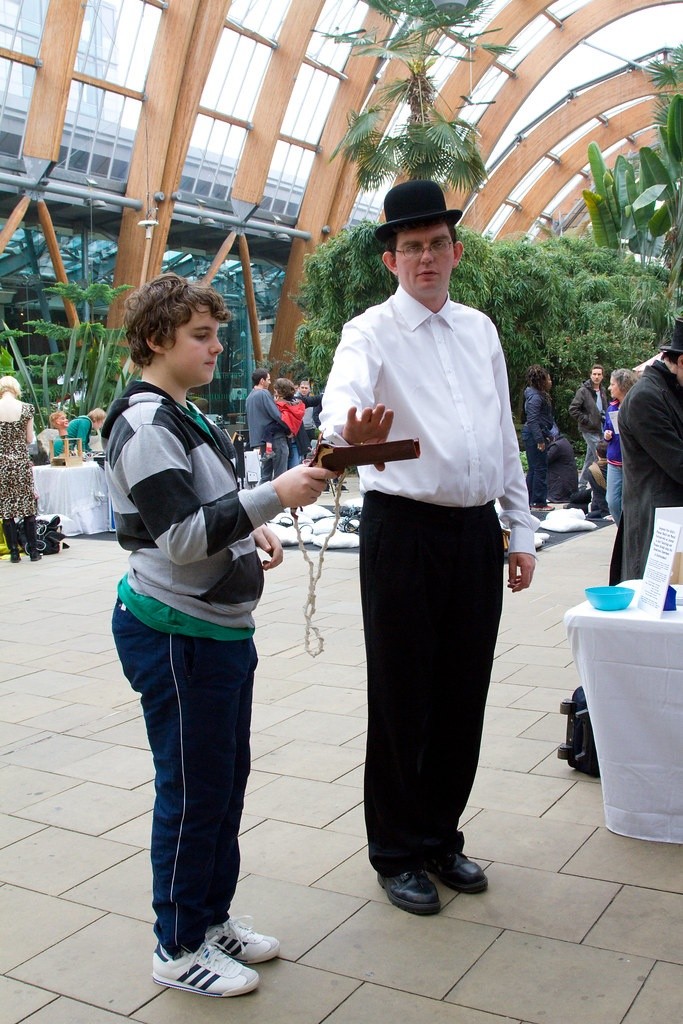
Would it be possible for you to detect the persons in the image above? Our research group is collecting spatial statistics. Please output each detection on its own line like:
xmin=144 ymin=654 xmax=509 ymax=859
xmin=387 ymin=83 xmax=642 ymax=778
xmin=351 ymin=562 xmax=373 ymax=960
xmin=53 ymin=407 xmax=107 ymax=460
xmin=44 ymin=411 xmax=78 ymax=457
xmin=568 ymin=364 xmax=607 ymax=490
xmin=602 ymin=367 xmax=637 ymax=526
xmin=584 ymin=439 xmax=609 ymax=517
xmin=0 ymin=376 xmax=43 ymax=563
xmin=524 ymin=363 xmax=556 ymax=512
xmin=319 ymin=179 xmax=540 ymax=915
xmin=103 ymin=272 xmax=336 ymax=998
xmin=244 ymin=367 xmax=323 ymax=485
xmin=608 ymin=317 xmax=683 ymax=581
xmin=545 ymin=428 xmax=577 ymax=504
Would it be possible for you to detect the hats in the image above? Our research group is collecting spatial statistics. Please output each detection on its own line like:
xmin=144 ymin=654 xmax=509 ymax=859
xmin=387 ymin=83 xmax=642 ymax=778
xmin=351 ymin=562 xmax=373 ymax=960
xmin=375 ymin=179 xmax=463 ymax=243
xmin=660 ymin=318 xmax=683 ymax=353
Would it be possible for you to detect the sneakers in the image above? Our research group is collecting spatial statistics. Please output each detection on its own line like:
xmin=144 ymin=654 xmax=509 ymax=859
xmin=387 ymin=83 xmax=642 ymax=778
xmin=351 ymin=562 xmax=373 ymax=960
xmin=204 ymin=914 xmax=280 ymax=964
xmin=532 ymin=505 xmax=555 ymax=511
xmin=153 ymin=937 xmax=258 ymax=997
xmin=260 ymin=451 xmax=275 ymax=461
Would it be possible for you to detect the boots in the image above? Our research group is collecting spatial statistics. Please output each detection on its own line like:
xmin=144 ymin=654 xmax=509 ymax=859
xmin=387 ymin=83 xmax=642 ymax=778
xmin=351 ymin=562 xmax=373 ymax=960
xmin=23 ymin=522 xmax=42 ymax=561
xmin=3 ymin=523 xmax=21 ymax=563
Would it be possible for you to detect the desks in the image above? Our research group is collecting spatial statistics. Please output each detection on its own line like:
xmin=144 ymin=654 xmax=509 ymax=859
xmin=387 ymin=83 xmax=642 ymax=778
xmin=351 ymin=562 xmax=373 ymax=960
xmin=33 ymin=462 xmax=113 ymax=540
xmin=566 ymin=579 xmax=683 ymax=842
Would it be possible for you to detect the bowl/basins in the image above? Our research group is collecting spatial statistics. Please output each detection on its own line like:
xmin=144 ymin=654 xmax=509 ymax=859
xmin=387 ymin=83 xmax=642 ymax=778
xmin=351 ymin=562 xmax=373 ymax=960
xmin=585 ymin=586 xmax=635 ymax=610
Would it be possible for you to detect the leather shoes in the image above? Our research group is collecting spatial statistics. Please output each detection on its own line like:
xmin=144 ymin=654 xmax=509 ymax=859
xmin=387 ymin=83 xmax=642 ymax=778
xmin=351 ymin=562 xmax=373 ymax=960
xmin=377 ymin=869 xmax=441 ymax=914
xmin=428 ymin=852 xmax=488 ymax=892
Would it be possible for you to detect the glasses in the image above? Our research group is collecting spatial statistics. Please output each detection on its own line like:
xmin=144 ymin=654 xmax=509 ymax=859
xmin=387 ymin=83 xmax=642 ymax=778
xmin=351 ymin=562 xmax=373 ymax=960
xmin=392 ymin=240 xmax=453 ymax=258
